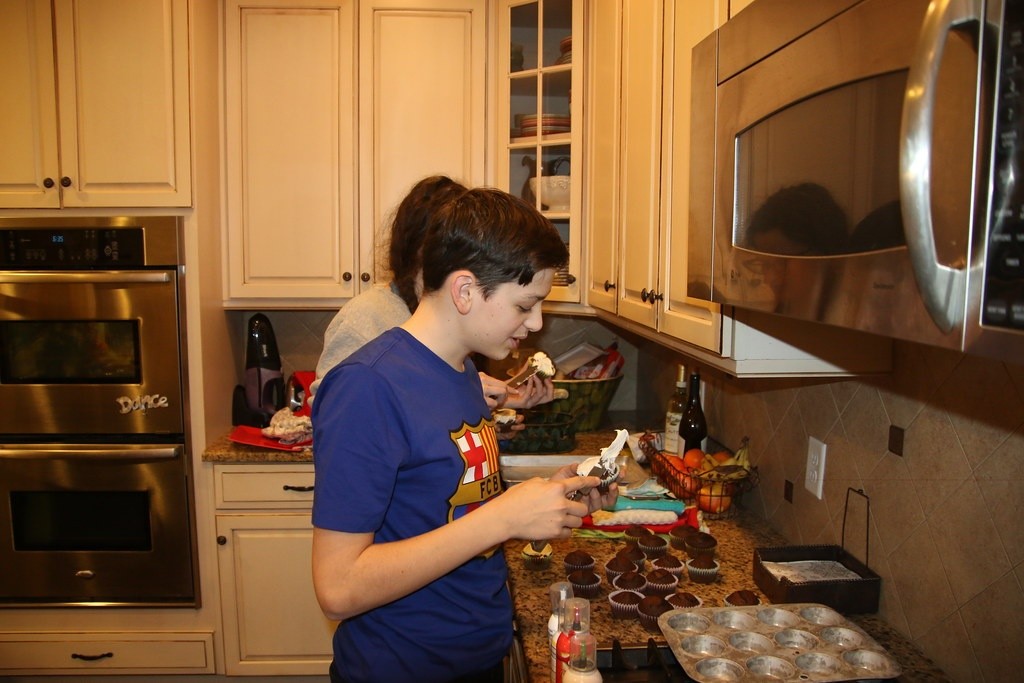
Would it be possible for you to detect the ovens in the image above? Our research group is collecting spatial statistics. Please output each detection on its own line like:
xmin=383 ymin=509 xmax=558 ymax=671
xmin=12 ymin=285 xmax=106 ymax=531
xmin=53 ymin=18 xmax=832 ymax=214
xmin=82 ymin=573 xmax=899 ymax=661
xmin=0 ymin=213 xmax=204 ymax=614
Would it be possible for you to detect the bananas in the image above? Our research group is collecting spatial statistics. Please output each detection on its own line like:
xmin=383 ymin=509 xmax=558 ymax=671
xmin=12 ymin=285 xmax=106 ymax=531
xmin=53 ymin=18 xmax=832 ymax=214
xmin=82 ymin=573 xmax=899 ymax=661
xmin=692 ymin=435 xmax=751 ymax=484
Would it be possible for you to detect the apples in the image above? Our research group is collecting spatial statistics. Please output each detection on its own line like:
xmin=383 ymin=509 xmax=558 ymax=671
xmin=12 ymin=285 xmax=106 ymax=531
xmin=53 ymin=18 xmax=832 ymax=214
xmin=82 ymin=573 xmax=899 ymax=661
xmin=651 ymin=448 xmax=733 ymax=513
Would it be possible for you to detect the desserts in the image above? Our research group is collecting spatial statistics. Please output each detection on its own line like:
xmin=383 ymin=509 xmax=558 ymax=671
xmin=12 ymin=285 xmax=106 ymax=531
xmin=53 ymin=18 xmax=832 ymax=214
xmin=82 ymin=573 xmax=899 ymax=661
xmin=490 ymin=408 xmax=517 ymax=433
xmin=525 ymin=351 xmax=556 ymax=388
xmin=577 ymin=456 xmax=620 ymax=495
xmin=521 ymin=524 xmax=762 ymax=631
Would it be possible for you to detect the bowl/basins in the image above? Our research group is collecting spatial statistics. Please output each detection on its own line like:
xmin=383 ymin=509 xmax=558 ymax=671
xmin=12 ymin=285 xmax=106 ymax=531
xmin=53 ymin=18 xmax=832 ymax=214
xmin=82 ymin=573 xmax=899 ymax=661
xmin=527 ymin=175 xmax=573 ymax=214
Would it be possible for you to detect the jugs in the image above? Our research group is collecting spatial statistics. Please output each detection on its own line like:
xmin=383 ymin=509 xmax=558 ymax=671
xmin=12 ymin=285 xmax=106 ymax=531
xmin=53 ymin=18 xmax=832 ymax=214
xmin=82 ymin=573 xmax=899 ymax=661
xmin=519 ymin=155 xmax=571 ymax=212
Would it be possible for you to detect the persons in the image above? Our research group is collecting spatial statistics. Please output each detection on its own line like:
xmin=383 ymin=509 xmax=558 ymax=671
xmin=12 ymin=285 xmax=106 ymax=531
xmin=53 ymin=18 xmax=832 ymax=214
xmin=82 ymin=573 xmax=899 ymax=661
xmin=307 ymin=175 xmax=469 ymax=409
xmin=744 ymin=183 xmax=903 ymax=257
xmin=308 ymin=189 xmax=618 ymax=683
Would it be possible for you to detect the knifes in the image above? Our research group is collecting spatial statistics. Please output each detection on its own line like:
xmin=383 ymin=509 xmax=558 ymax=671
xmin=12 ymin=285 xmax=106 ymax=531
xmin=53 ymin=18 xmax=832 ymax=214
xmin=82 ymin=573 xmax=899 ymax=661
xmin=489 ymin=364 xmax=539 ymax=401
xmin=532 ymin=429 xmax=629 ymax=553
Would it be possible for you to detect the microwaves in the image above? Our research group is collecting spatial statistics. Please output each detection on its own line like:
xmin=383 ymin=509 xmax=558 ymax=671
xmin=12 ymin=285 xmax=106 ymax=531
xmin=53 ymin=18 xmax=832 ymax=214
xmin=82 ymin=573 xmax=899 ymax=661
xmin=685 ymin=0 xmax=1024 ymax=441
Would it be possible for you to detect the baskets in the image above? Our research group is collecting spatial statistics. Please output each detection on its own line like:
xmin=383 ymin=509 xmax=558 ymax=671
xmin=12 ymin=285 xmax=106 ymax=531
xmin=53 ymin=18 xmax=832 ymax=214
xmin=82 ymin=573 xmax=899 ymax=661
xmin=638 ymin=428 xmax=761 ymax=521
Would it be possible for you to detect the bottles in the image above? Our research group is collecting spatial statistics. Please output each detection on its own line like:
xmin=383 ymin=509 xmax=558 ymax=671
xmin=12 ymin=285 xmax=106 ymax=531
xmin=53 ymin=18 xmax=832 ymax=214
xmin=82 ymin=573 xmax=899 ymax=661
xmin=677 ymin=371 xmax=709 ymax=460
xmin=562 ymin=631 xmax=604 ymax=683
xmin=664 ymin=363 xmax=690 ymax=458
xmin=554 ymin=598 xmax=592 ymax=683
xmin=547 ymin=581 xmax=575 ymax=656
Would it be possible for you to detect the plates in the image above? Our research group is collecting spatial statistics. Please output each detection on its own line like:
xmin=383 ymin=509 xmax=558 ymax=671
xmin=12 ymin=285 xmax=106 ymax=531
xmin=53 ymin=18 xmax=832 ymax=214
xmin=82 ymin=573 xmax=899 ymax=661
xmin=555 ymin=35 xmax=573 ymax=66
xmin=550 ymin=242 xmax=572 ymax=287
xmin=511 ymin=111 xmax=570 ymax=141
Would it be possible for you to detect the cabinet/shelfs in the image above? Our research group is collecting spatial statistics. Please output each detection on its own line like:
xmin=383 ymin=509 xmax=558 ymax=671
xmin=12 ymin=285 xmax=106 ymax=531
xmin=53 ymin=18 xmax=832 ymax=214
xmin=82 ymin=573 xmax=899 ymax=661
xmin=1 ymin=1 xmax=886 ymax=387
